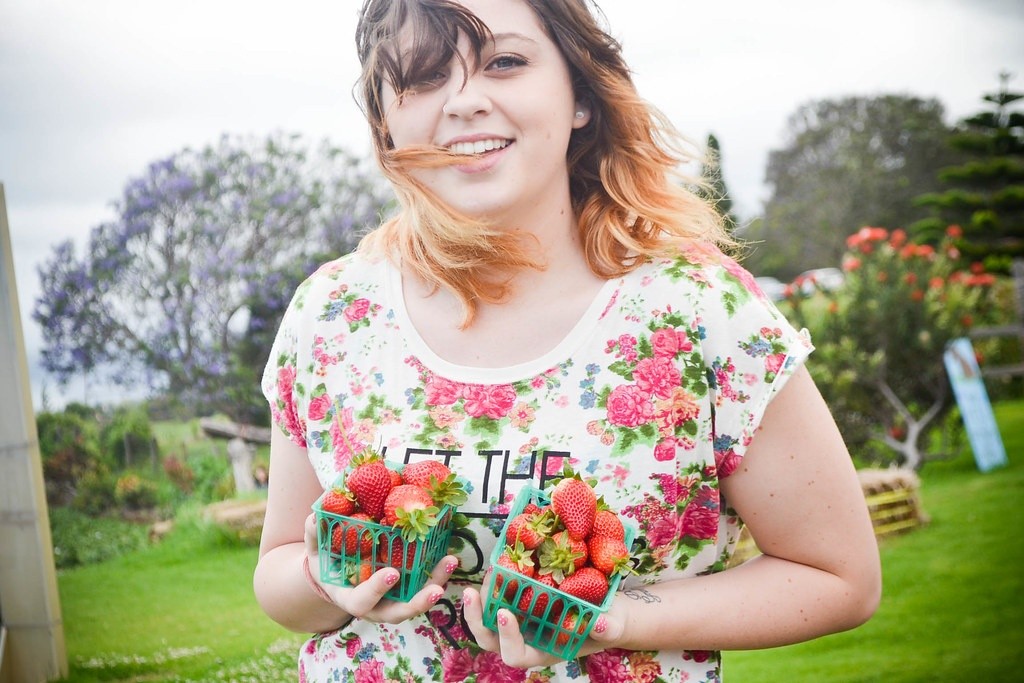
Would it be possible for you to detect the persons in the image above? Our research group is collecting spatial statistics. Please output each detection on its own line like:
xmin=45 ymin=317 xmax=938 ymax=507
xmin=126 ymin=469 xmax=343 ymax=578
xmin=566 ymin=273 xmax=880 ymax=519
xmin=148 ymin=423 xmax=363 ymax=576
xmin=254 ymin=0 xmax=883 ymax=683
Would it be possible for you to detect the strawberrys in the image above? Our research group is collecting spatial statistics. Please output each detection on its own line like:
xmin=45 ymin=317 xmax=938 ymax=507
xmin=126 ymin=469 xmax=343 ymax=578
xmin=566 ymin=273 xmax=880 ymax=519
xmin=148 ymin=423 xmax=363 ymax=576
xmin=319 ymin=434 xmax=468 ymax=588
xmin=489 ymin=463 xmax=630 ymax=645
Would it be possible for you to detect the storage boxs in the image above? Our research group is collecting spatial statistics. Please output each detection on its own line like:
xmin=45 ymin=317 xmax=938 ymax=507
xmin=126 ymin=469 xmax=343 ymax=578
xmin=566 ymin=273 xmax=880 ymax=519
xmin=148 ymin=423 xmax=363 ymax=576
xmin=856 ymin=467 xmax=932 ymax=539
